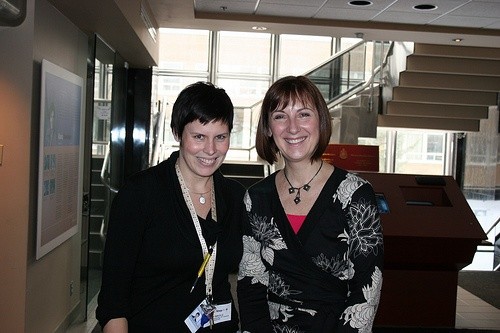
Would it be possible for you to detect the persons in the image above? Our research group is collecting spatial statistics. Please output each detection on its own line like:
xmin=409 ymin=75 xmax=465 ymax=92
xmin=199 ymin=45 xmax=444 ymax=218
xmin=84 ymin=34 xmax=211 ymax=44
xmin=96 ymin=81 xmax=249 ymax=333
xmin=236 ymin=76 xmax=385 ymax=333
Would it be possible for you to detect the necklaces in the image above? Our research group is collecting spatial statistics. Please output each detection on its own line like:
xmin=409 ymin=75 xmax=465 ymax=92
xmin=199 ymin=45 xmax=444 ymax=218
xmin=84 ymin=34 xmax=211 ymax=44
xmin=284 ymin=159 xmax=324 ymax=205
xmin=186 ymin=188 xmax=212 ymax=205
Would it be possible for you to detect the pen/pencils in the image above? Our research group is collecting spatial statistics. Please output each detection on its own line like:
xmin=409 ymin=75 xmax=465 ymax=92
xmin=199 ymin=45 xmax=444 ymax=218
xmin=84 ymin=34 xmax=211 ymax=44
xmin=189 ymin=248 xmax=213 ymax=295
